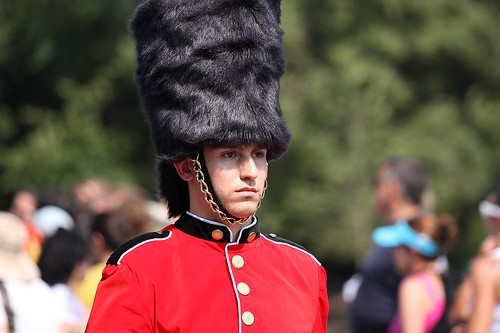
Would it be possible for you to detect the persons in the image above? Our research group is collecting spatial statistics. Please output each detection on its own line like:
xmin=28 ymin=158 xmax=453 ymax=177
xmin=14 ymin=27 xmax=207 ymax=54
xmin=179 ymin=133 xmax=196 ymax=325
xmin=444 ymin=235 xmax=496 ymax=332
xmin=349 ymin=153 xmax=429 ymax=332
xmin=466 ymin=189 xmax=500 ymax=333
xmin=83 ymin=0 xmax=335 ymax=333
xmin=370 ymin=213 xmax=458 ymax=333
xmin=0 ymin=173 xmax=171 ymax=333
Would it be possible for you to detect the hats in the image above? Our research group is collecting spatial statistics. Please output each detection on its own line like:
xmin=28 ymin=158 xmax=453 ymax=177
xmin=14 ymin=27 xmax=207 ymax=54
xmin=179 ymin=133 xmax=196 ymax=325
xmin=0 ymin=213 xmax=45 ymax=282
xmin=28 ymin=206 xmax=76 ymax=248
xmin=131 ymin=0 xmax=291 ymax=219
xmin=371 ymin=220 xmax=438 ymax=255
